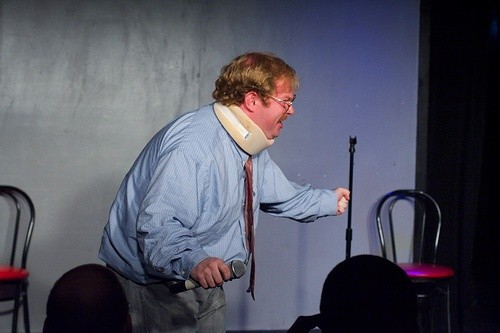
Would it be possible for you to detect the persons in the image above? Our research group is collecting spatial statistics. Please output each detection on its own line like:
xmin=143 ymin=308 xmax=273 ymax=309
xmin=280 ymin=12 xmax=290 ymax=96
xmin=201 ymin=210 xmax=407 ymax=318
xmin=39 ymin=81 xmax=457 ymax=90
xmin=286 ymin=255 xmax=418 ymax=333
xmin=43 ymin=264 xmax=132 ymax=333
xmin=98 ymin=52 xmax=350 ymax=333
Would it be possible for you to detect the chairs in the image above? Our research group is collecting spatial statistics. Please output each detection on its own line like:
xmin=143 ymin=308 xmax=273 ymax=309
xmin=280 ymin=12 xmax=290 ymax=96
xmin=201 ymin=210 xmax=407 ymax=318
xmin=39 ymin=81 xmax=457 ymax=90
xmin=376 ymin=190 xmax=454 ymax=333
xmin=0 ymin=185 xmax=35 ymax=333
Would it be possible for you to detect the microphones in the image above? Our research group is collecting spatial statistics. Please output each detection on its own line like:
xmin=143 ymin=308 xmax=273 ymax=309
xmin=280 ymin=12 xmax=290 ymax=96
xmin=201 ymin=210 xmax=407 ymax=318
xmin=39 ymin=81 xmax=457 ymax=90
xmin=170 ymin=259 xmax=246 ymax=295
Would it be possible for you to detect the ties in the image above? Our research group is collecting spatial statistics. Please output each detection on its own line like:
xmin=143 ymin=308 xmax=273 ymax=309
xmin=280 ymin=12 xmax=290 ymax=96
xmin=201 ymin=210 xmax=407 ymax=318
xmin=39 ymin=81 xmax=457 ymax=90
xmin=245 ymin=156 xmax=256 ymax=301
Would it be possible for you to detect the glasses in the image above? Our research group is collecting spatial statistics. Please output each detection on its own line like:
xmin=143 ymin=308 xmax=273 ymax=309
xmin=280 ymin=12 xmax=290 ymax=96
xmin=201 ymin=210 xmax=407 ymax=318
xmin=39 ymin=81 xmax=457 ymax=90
xmin=247 ymin=87 xmax=297 ymax=106
